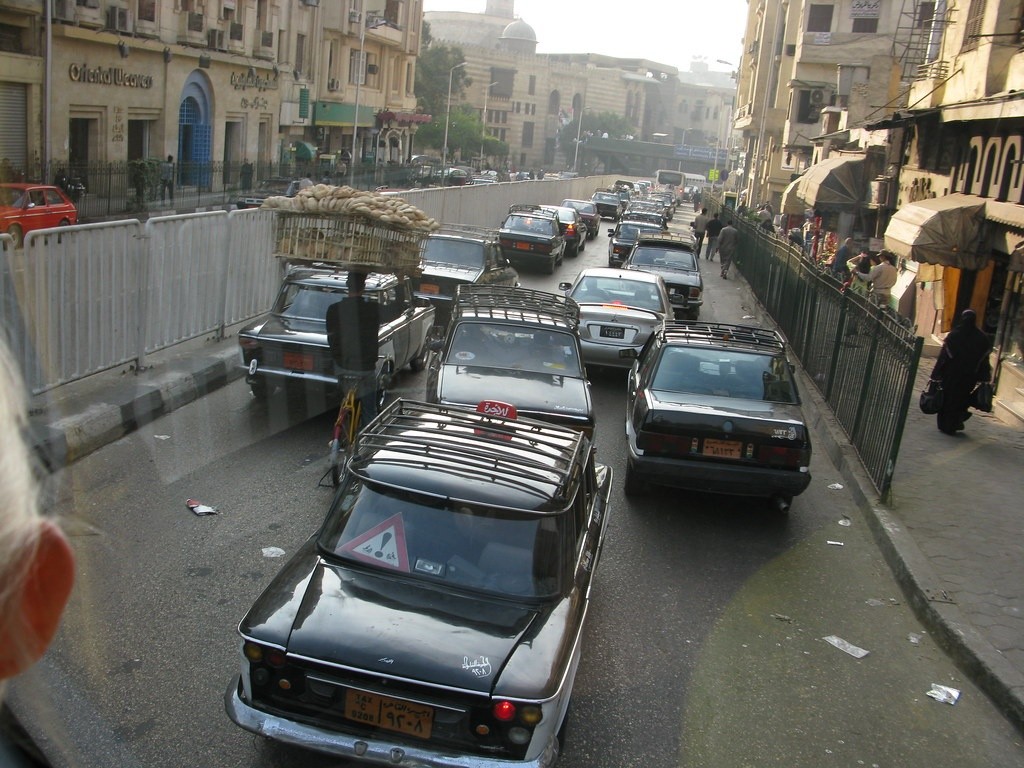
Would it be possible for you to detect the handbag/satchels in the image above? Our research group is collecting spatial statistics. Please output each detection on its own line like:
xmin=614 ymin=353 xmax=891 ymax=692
xmin=920 ymin=381 xmax=941 ymax=414
xmin=968 ymin=383 xmax=993 ymax=412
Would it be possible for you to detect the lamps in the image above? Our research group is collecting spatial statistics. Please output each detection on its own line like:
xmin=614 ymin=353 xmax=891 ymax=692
xmin=183 ymin=45 xmax=210 ymax=68
xmin=278 ymin=61 xmax=299 ymax=81
xmin=144 ymin=36 xmax=173 ymax=64
xmin=97 ymin=29 xmax=128 ymax=58
xmin=231 ymin=54 xmax=257 ymax=76
xmin=772 ymin=142 xmax=788 ymax=153
xmin=257 ymin=58 xmax=280 ymax=76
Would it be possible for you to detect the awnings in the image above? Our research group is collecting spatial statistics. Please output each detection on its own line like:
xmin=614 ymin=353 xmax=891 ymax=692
xmin=883 ymin=193 xmax=990 ymax=271
xmin=296 ymin=140 xmax=317 ymax=161
xmin=780 ymin=176 xmax=811 ymax=215
xmin=796 ymin=154 xmax=866 ymax=213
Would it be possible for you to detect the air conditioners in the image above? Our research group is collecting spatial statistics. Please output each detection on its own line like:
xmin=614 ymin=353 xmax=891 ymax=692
xmin=350 ymin=11 xmax=363 ymax=22
xmin=304 ymin=0 xmax=321 ymax=6
xmin=810 ymin=89 xmax=833 ymax=105
xmin=367 ymin=16 xmax=379 ymax=28
xmin=330 ymin=79 xmax=340 ymax=91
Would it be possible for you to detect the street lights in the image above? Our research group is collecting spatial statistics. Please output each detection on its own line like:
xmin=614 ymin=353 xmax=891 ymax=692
xmin=571 ymin=106 xmax=593 ymax=172
xmin=678 ymin=127 xmax=694 ymax=172
xmin=439 ymin=60 xmax=469 ymax=187
xmin=349 ymin=20 xmax=387 ymax=187
xmin=480 ymin=81 xmax=498 ymax=170
xmin=707 ymin=89 xmax=726 ymax=196
xmin=716 ymin=59 xmax=739 ymax=199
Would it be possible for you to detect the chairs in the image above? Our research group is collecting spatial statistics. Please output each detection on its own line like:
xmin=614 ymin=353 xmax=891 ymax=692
xmin=533 ymin=334 xmax=550 ymax=343
xmin=357 ymin=513 xmax=411 ymax=550
xmin=479 ymin=543 xmax=533 ymax=573
xmin=666 ymin=353 xmax=765 ymax=396
xmin=634 ymin=290 xmax=652 ymax=302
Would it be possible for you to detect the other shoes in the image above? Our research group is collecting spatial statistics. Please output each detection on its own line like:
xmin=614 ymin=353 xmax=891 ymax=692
xmin=721 ymin=273 xmax=726 ymax=279
xmin=710 ymin=258 xmax=713 ymax=261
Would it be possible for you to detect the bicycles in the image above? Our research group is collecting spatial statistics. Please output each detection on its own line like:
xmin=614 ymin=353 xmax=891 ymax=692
xmin=317 ymin=354 xmax=392 ymax=491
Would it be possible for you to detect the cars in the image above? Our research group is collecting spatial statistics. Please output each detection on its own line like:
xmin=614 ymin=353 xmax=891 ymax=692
xmin=0 ymin=182 xmax=78 ymax=250
xmin=232 ymin=258 xmax=438 ymax=415
xmin=410 ymin=153 xmax=706 ymax=324
xmin=423 ymin=285 xmax=597 ymax=442
xmin=559 ymin=265 xmax=686 ymax=371
xmin=618 ymin=318 xmax=812 ymax=516
xmin=221 ymin=396 xmax=616 ymax=768
xmin=235 ymin=175 xmax=301 ymax=210
xmin=411 ymin=222 xmax=521 ymax=334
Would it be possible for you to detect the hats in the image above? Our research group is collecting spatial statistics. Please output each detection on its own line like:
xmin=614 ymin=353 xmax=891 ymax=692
xmin=876 ymin=249 xmax=891 ymax=257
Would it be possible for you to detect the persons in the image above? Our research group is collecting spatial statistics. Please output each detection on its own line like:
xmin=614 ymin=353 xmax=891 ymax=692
xmin=475 ymin=162 xmax=545 ymax=181
xmin=572 ymin=129 xmax=630 ymax=143
xmin=0 ymin=262 xmax=112 ymax=768
xmin=376 ymin=158 xmax=384 ymax=185
xmin=691 ymin=189 xmax=702 ymax=212
xmin=577 ymin=277 xmax=609 ymax=301
xmin=240 ymin=158 xmax=254 ymax=195
xmin=621 ymin=225 xmax=635 ymax=237
xmin=133 ymin=162 xmax=147 ymax=205
xmin=690 ymin=208 xmax=739 ymax=279
xmin=386 ymin=159 xmax=418 ymax=188
xmin=54 ymin=166 xmax=72 ymax=193
xmin=160 ymin=154 xmax=177 ymax=210
xmin=0 ymin=158 xmax=16 ymax=207
xmin=928 ymin=311 xmax=993 ymax=435
xmin=634 ymin=249 xmax=654 ymax=264
xmin=298 ymin=171 xmax=332 ymax=191
xmin=326 ymin=271 xmax=380 ymax=428
xmin=334 ymin=158 xmax=347 ymax=187
xmin=736 ymin=201 xmax=897 ymax=337
